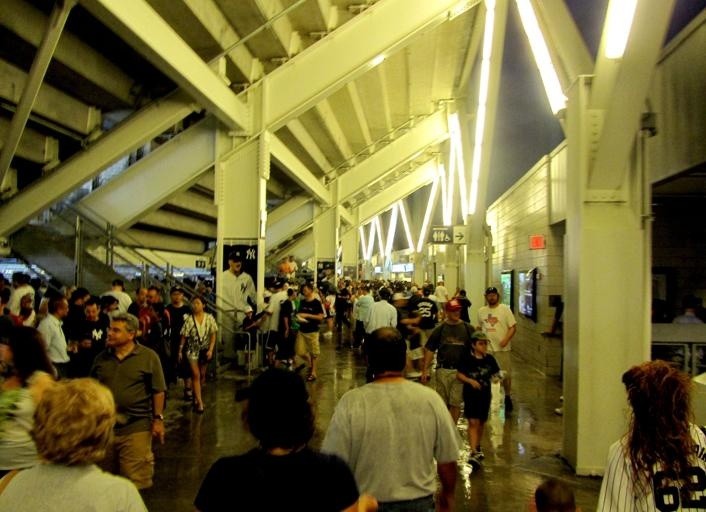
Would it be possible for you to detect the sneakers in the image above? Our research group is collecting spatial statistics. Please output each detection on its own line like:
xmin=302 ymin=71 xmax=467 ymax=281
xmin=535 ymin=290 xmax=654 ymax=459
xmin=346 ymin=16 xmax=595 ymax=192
xmin=294 ymin=364 xmax=315 ymax=382
xmin=469 ymin=446 xmax=484 ymax=467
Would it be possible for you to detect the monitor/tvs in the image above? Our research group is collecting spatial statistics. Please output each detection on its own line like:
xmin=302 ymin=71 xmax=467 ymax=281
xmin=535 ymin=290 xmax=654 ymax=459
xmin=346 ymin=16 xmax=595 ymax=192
xmin=518 ymin=268 xmax=536 ymax=324
xmin=501 ymin=270 xmax=514 ymax=314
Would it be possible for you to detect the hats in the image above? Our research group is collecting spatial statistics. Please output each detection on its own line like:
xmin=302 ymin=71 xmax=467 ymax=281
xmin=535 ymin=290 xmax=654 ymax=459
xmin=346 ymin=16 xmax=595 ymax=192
xmin=472 ymin=332 xmax=489 ymax=340
xmin=446 ymin=300 xmax=461 ymax=311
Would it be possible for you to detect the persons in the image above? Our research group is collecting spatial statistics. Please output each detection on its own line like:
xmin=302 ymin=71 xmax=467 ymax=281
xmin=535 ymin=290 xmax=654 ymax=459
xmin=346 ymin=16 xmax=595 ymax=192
xmin=673 ymin=298 xmax=704 ymax=324
xmin=595 ymin=360 xmax=705 ymax=512
xmin=0 ymin=254 xmax=519 ymax=511
xmin=529 ymin=476 xmax=581 ymax=512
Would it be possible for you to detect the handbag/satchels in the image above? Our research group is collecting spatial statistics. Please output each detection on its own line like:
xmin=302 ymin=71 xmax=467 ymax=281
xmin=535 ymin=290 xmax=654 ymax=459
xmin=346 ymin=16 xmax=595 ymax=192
xmin=198 ymin=349 xmax=208 ymax=364
